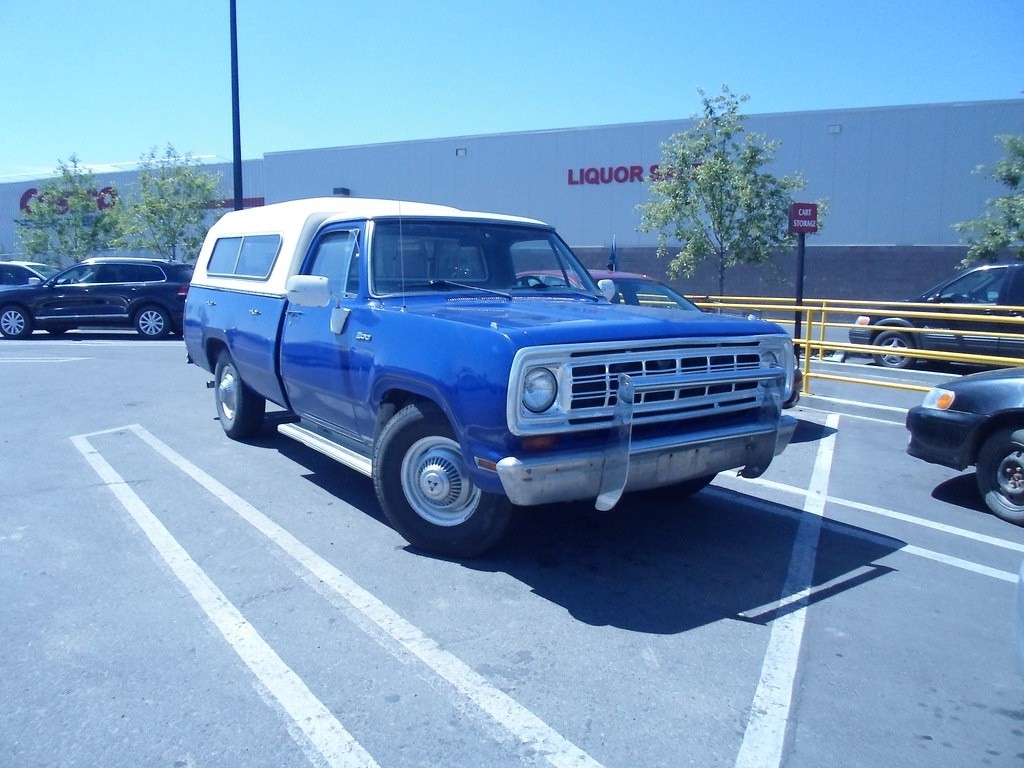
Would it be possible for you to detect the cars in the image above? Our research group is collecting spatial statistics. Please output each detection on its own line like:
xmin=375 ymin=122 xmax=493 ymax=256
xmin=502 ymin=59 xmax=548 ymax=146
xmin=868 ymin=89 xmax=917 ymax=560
xmin=513 ymin=270 xmax=804 ymax=408
xmin=905 ymin=367 xmax=1024 ymax=524
xmin=0 ymin=261 xmax=75 ymax=285
xmin=848 ymin=263 xmax=1024 ymax=369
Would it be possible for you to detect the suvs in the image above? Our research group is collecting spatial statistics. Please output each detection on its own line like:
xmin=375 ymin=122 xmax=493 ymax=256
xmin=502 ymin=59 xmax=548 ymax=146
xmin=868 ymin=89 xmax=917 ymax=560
xmin=182 ymin=193 xmax=799 ymax=557
xmin=0 ymin=257 xmax=195 ymax=340
